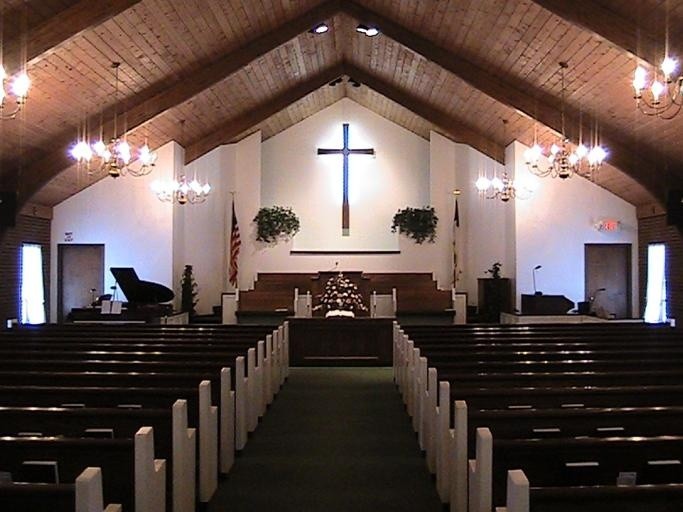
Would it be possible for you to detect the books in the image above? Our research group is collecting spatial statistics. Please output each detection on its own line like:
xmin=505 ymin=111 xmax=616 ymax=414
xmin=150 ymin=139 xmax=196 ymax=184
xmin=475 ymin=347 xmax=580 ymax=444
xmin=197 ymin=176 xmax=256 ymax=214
xmin=110 ymin=301 xmax=123 ymax=315
xmin=100 ymin=300 xmax=110 ymax=315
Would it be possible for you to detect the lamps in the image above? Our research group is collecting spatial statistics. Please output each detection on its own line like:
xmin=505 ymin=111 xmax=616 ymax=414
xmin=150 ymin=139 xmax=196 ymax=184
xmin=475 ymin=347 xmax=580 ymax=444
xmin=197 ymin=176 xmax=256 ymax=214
xmin=149 ymin=119 xmax=211 ymax=202
xmin=630 ymin=3 xmax=683 ymax=121
xmin=524 ymin=62 xmax=613 ymax=182
xmin=69 ymin=62 xmax=158 ymax=179
xmin=0 ymin=64 xmax=32 ymax=122
xmin=477 ymin=118 xmax=535 ymax=201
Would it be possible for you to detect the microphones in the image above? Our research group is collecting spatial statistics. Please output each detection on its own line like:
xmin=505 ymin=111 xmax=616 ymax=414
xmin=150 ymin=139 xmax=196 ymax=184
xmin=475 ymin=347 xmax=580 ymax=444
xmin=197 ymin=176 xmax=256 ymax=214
xmin=590 ymin=288 xmax=606 ymax=307
xmin=533 ymin=265 xmax=542 ymax=295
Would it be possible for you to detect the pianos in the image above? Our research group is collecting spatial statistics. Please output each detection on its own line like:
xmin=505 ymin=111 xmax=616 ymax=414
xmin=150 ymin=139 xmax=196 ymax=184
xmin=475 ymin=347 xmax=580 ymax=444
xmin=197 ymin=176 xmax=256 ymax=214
xmin=71 ymin=267 xmax=175 ymax=321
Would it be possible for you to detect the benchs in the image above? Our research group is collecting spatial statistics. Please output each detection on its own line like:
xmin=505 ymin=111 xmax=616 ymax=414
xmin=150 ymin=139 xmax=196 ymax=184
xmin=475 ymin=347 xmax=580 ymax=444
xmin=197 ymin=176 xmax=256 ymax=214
xmin=391 ymin=319 xmax=683 ymax=512
xmin=0 ymin=317 xmax=288 ymax=512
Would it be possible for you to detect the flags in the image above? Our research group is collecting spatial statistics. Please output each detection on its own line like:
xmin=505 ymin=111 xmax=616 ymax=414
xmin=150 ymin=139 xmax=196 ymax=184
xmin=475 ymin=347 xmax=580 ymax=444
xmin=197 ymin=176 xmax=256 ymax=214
xmin=228 ymin=198 xmax=243 ymax=289
xmin=451 ymin=198 xmax=464 ymax=287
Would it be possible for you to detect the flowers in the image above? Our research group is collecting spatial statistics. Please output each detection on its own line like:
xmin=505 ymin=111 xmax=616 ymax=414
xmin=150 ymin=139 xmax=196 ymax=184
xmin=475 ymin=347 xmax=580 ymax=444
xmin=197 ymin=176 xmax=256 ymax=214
xmin=320 ymin=273 xmax=368 ymax=313
